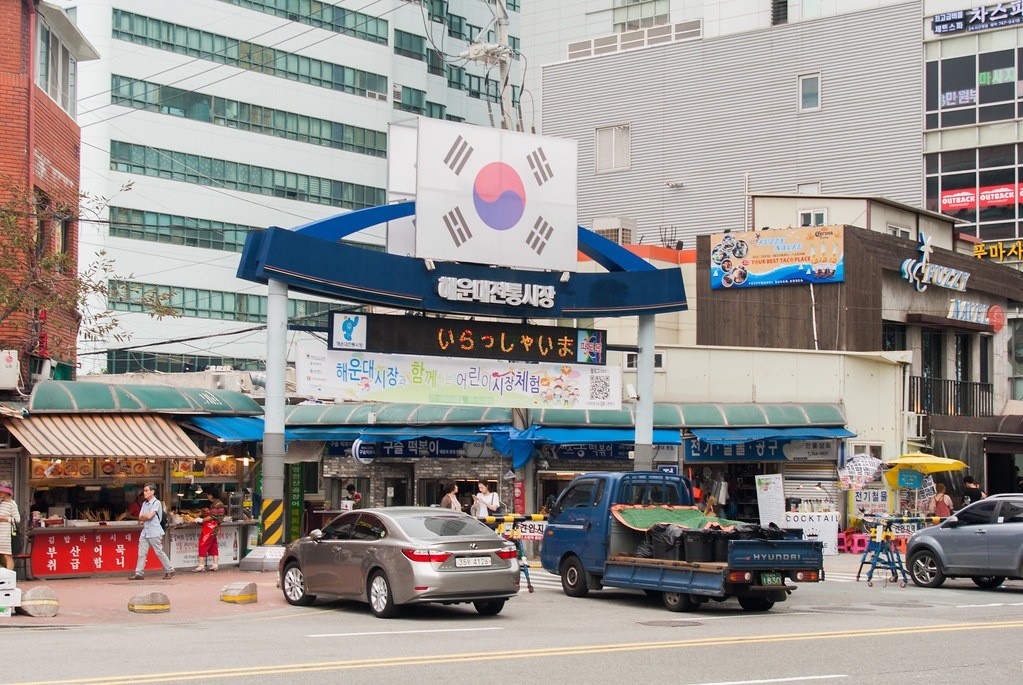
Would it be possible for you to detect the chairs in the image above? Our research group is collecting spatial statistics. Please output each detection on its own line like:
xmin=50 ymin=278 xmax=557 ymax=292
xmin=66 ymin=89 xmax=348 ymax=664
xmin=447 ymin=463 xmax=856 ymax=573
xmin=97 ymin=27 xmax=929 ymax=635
xmin=13 ymin=537 xmax=32 ymax=580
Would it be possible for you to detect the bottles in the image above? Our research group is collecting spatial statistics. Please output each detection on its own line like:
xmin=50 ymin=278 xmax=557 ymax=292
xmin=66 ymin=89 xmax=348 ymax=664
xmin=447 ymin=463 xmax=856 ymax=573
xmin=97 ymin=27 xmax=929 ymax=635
xmin=32 ymin=517 xmax=36 ymax=527
xmin=803 ymin=497 xmax=835 ymax=513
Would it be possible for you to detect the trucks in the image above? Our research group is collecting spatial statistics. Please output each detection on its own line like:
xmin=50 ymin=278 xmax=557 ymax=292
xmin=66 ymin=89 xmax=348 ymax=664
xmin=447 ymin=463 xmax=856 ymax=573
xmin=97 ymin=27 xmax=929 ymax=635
xmin=540 ymin=471 xmax=824 ymax=612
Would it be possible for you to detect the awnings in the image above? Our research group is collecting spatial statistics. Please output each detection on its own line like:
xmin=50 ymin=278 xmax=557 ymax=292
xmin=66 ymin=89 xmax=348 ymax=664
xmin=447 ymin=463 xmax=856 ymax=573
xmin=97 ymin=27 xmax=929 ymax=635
xmin=4 ymin=416 xmax=857 ymax=460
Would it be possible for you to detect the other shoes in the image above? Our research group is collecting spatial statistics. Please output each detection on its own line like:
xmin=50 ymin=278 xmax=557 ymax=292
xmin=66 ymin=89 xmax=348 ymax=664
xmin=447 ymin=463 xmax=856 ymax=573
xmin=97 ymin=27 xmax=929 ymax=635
xmin=128 ymin=572 xmax=145 ymax=580
xmin=192 ymin=566 xmax=205 ymax=573
xmin=209 ymin=565 xmax=218 ymax=571
xmin=163 ymin=570 xmax=175 ymax=579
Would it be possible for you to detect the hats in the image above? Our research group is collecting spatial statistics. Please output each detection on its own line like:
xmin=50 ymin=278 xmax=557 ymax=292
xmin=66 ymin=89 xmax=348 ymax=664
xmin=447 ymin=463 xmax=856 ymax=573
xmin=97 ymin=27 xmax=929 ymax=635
xmin=0 ymin=480 xmax=13 ymax=496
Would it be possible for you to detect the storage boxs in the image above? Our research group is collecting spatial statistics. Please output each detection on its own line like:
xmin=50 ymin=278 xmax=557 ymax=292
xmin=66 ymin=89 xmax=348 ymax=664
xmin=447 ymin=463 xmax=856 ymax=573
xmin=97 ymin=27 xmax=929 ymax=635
xmin=0 ymin=607 xmax=12 ymax=618
xmin=0 ymin=567 xmax=17 ymax=590
xmin=0 ymin=588 xmax=21 ymax=607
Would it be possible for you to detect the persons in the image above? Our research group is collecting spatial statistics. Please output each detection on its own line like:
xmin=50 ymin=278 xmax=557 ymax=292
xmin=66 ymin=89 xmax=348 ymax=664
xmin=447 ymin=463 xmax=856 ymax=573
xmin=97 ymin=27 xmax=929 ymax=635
xmin=346 ymin=485 xmax=360 ymax=510
xmin=128 ymin=482 xmax=175 ymax=581
xmin=471 ymin=479 xmax=500 ymax=531
xmin=191 ymin=490 xmax=224 ymax=573
xmin=0 ymin=481 xmax=20 ymax=570
xmin=440 ymin=482 xmax=462 ymax=511
xmin=962 ymin=476 xmax=985 ymax=507
xmin=927 ymin=484 xmax=953 ymax=517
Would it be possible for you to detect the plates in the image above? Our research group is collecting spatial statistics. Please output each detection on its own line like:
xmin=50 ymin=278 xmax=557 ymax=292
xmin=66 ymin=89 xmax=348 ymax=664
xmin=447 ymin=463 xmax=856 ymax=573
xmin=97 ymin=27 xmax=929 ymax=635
xmin=712 ymin=235 xmax=748 ymax=288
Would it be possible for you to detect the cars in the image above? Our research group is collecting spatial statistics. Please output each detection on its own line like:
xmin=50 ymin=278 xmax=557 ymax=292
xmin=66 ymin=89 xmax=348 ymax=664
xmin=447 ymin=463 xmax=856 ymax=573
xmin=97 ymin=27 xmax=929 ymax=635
xmin=906 ymin=494 xmax=1023 ymax=589
xmin=279 ymin=506 xmax=521 ymax=618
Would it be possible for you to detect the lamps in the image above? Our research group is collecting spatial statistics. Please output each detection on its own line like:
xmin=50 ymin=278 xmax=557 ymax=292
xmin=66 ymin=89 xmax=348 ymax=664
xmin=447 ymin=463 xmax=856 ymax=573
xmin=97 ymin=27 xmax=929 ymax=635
xmin=815 ymin=482 xmax=833 ymax=502
xmin=177 ymin=490 xmax=184 ymax=496
xmin=196 ymin=485 xmax=203 ymax=494
xmin=50 ymin=458 xmax=54 ymax=463
xmin=676 ymin=240 xmax=683 ymax=250
xmin=236 ymin=443 xmax=255 ymax=466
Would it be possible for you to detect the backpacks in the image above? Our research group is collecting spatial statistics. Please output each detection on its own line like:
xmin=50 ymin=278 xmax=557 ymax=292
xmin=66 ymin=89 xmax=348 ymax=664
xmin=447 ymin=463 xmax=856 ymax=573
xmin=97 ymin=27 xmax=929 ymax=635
xmin=152 ymin=500 xmax=167 ymax=530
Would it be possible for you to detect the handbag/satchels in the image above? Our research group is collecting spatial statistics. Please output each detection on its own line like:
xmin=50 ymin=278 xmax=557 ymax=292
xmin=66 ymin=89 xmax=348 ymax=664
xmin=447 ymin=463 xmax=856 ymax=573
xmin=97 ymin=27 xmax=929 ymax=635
xmin=487 ymin=492 xmax=504 ymax=524
xmin=11 ymin=519 xmax=21 ymax=554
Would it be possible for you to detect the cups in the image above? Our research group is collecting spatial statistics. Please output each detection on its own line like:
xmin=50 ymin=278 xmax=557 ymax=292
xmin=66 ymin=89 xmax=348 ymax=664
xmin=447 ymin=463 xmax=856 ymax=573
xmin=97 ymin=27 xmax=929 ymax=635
xmin=791 ymin=504 xmax=796 ymax=512
xmin=798 ymin=504 xmax=805 ymax=513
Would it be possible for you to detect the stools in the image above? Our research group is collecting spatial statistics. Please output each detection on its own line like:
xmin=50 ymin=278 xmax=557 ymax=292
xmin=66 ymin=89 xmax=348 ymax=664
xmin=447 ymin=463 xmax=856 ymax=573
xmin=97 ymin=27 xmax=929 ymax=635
xmin=838 ymin=533 xmax=847 ymax=553
xmin=850 ymin=534 xmax=867 ymax=554
xmin=889 ymin=538 xmax=905 ymax=554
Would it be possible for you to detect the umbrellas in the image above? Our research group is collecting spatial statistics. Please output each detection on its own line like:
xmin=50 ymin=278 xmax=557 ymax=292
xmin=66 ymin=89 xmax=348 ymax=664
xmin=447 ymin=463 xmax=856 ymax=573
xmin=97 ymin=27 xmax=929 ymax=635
xmin=885 ymin=451 xmax=970 ymax=514
